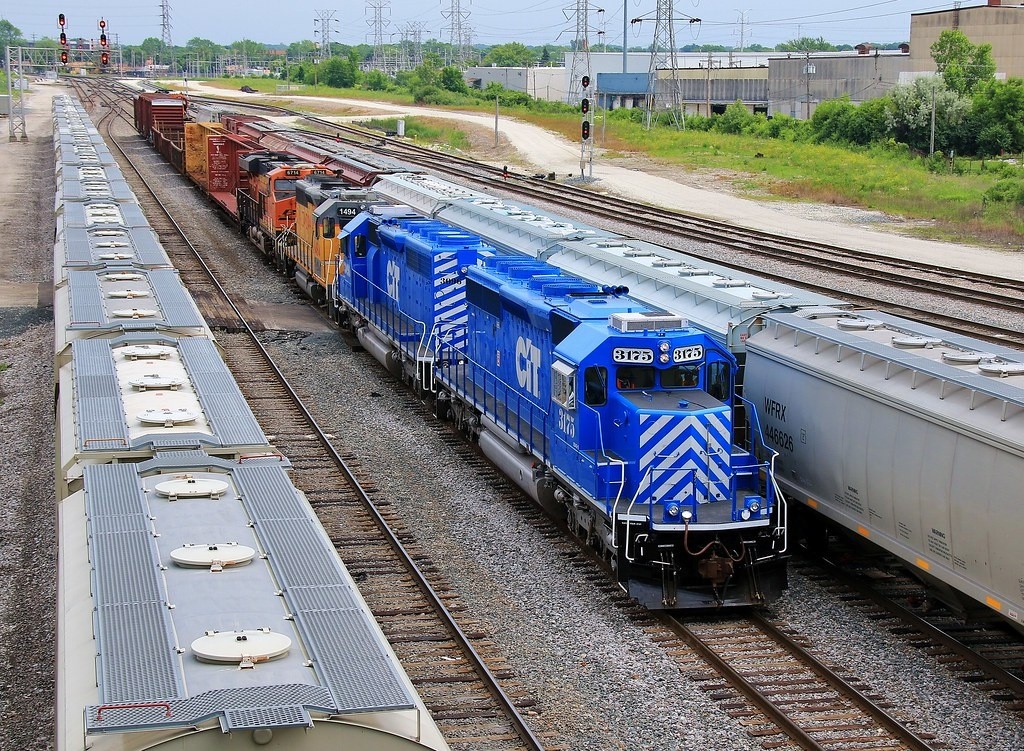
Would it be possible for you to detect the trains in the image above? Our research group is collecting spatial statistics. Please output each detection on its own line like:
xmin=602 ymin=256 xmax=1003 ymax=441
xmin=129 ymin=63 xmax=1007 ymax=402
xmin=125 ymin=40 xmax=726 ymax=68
xmin=49 ymin=95 xmax=447 ymax=751
xmin=192 ymin=98 xmax=1024 ymax=629
xmin=135 ymin=94 xmax=792 ymax=620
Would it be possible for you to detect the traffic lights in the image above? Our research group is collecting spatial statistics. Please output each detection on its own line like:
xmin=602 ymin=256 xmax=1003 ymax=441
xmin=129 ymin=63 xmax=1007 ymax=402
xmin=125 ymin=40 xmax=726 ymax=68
xmin=62 ymin=52 xmax=68 ymax=63
xmin=102 ymin=53 xmax=108 ymax=65
xmin=100 ymin=21 xmax=105 ymax=28
xmin=582 ymin=121 xmax=589 ymax=140
xmin=61 ymin=33 xmax=66 ymax=45
xmin=101 ymin=34 xmax=106 ymax=46
xmin=582 ymin=99 xmax=589 ymax=114
xmin=59 ymin=14 xmax=65 ymax=25
xmin=582 ymin=76 xmax=590 ymax=88
